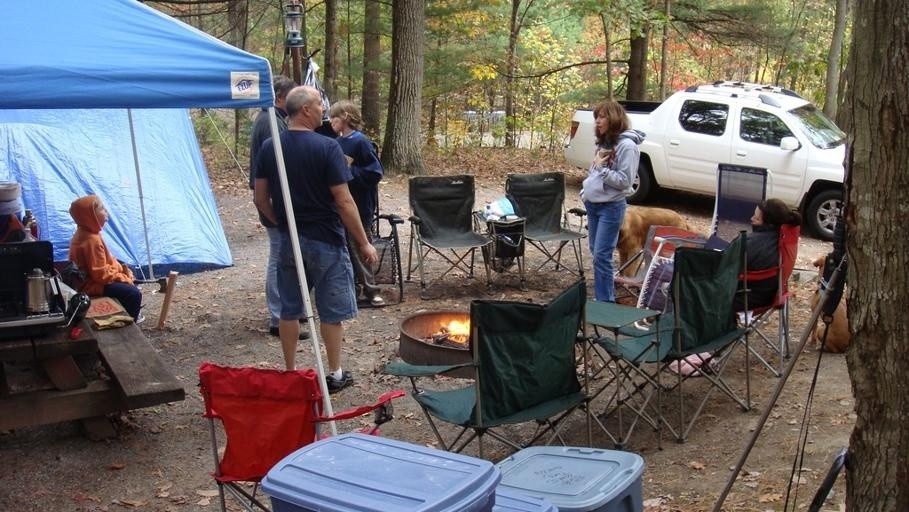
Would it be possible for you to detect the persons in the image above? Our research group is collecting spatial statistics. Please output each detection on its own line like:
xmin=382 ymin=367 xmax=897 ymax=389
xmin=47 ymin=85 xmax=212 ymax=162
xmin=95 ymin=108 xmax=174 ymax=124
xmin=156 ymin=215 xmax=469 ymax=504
xmin=249 ymin=74 xmax=316 ymax=340
xmin=732 ymin=197 xmax=802 ymax=310
xmin=578 ymin=100 xmax=646 ymax=304
xmin=328 ymin=100 xmax=387 ymax=308
xmin=1 ymin=197 xmax=38 ymax=245
xmin=68 ymin=195 xmax=142 ymax=323
xmin=254 ymin=84 xmax=379 ymax=395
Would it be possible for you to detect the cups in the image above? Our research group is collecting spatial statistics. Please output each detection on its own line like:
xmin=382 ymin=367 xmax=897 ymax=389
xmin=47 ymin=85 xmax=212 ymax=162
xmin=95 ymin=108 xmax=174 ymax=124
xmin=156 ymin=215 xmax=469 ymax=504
xmin=27 ymin=276 xmax=54 ymax=313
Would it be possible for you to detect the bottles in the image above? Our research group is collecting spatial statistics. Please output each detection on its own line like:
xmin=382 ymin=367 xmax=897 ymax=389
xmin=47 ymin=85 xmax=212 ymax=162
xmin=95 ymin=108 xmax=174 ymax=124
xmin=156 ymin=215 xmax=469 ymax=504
xmin=22 ymin=209 xmax=38 ymax=241
xmin=486 ymin=206 xmax=491 ymax=218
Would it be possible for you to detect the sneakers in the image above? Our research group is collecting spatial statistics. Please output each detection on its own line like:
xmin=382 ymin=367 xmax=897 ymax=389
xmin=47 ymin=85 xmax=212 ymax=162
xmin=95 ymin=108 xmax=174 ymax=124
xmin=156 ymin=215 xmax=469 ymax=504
xmin=269 ymin=326 xmax=310 ymax=339
xmin=371 ymin=297 xmax=386 ymax=307
xmin=326 ymin=371 xmax=353 ymax=394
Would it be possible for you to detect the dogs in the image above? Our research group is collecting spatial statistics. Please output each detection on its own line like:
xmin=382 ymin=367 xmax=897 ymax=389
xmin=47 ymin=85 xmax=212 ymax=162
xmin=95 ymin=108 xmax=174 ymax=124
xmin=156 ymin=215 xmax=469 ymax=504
xmin=810 ymin=256 xmax=849 ymax=353
xmin=585 ymin=205 xmax=708 ymax=277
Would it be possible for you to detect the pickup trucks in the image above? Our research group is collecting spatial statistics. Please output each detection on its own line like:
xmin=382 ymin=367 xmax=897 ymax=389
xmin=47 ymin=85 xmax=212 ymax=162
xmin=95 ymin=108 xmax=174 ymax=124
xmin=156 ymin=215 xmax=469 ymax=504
xmin=563 ymin=81 xmax=848 ymax=241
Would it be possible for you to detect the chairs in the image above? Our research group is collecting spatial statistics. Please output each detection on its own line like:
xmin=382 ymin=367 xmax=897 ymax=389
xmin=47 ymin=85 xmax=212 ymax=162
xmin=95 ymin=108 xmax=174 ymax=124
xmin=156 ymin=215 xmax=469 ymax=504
xmin=358 ymin=185 xmax=404 ymax=307
xmin=406 ymin=173 xmax=495 ymax=294
xmin=503 ymin=171 xmax=587 ymax=287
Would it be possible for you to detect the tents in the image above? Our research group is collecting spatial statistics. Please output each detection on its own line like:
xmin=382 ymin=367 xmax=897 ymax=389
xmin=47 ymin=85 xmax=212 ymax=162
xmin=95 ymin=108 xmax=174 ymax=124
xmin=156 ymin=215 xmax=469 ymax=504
xmin=0 ymin=109 xmax=236 ymax=270
